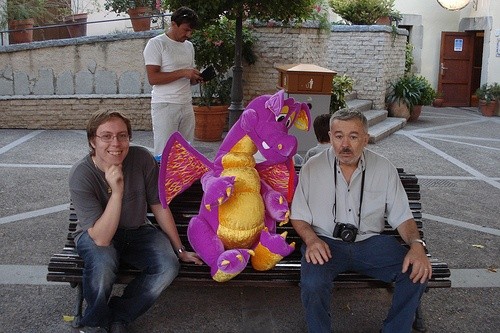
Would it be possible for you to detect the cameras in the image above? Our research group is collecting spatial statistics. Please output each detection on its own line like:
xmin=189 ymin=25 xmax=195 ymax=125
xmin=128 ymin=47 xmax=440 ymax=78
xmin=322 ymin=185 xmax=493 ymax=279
xmin=333 ymin=222 xmax=358 ymax=242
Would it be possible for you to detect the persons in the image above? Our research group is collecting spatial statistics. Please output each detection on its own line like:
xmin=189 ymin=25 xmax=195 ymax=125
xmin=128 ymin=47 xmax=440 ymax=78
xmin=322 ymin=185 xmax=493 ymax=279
xmin=67 ymin=108 xmax=202 ymax=333
xmin=302 ymin=114 xmax=331 ymax=164
xmin=143 ymin=7 xmax=204 ymax=158
xmin=289 ymin=108 xmax=432 ymax=333
xmin=294 ymin=154 xmax=303 ymax=164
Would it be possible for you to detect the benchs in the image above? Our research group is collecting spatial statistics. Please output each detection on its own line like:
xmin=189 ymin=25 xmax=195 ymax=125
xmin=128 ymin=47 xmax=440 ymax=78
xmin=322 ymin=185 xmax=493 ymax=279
xmin=46 ymin=164 xmax=453 ymax=333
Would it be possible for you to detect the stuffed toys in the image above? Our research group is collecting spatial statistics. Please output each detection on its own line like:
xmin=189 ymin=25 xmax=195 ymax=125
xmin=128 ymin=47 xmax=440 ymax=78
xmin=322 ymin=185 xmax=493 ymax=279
xmin=159 ymin=90 xmax=312 ymax=283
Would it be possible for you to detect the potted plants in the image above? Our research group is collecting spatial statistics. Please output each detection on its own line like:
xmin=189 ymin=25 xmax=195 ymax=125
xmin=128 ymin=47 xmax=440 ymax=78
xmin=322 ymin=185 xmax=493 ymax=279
xmin=477 ymin=81 xmax=500 ymax=116
xmin=0 ymin=0 xmax=56 ymax=44
xmin=55 ymin=8 xmax=87 ymax=37
xmin=386 ymin=75 xmax=436 ymax=122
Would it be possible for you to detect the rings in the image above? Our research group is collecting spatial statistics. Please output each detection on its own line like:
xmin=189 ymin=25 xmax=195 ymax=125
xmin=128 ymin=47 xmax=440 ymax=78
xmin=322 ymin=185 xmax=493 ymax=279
xmin=424 ymin=269 xmax=428 ymax=270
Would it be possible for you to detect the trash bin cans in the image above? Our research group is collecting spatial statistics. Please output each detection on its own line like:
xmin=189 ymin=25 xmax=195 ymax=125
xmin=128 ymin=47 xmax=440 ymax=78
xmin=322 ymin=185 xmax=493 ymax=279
xmin=271 ymin=63 xmax=337 ymax=159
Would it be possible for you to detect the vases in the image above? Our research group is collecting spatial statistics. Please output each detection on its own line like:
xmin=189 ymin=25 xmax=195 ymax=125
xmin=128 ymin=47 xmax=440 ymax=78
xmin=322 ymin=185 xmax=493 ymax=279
xmin=126 ymin=6 xmax=152 ymax=32
xmin=193 ymin=103 xmax=230 ymax=142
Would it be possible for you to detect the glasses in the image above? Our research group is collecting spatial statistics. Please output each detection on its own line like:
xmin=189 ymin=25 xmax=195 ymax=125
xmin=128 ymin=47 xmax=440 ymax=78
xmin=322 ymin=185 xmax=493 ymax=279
xmin=96 ymin=134 xmax=131 ymax=142
xmin=175 ymin=13 xmax=198 ymax=19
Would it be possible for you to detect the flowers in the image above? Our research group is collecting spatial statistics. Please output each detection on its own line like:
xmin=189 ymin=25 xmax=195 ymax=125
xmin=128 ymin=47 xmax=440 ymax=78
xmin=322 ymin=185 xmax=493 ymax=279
xmin=104 ymin=0 xmax=164 ymax=24
xmin=190 ymin=12 xmax=265 ymax=109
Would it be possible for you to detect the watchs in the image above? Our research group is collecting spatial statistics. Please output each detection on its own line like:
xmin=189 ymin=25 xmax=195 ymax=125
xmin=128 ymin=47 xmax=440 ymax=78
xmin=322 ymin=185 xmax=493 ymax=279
xmin=410 ymin=239 xmax=426 ymax=250
xmin=175 ymin=246 xmax=185 ymax=254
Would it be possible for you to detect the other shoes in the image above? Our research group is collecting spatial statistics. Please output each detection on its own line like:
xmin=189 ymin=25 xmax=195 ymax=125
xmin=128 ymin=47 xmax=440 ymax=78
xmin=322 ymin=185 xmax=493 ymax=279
xmin=79 ymin=326 xmax=106 ymax=333
xmin=106 ymin=295 xmax=130 ymax=333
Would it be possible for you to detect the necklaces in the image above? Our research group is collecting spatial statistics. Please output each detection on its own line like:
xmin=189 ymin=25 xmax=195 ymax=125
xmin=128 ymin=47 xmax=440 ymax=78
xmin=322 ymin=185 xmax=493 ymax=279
xmin=92 ymin=156 xmax=112 ymax=193
